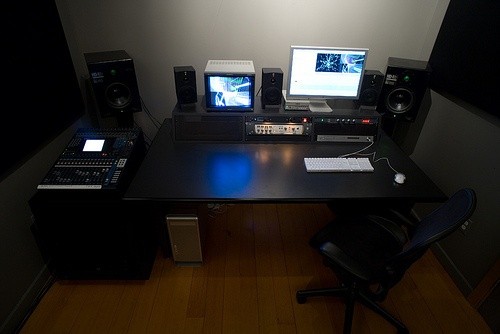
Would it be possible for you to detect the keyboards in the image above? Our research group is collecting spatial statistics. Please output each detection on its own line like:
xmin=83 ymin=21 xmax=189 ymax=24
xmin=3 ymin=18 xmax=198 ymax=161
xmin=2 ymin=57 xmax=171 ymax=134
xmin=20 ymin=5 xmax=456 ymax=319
xmin=304 ymin=157 xmax=375 ymax=173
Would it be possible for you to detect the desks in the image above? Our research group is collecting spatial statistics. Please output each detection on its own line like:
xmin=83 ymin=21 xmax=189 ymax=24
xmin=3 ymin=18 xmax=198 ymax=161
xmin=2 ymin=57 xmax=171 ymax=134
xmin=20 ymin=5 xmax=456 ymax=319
xmin=122 ymin=118 xmax=451 ymax=203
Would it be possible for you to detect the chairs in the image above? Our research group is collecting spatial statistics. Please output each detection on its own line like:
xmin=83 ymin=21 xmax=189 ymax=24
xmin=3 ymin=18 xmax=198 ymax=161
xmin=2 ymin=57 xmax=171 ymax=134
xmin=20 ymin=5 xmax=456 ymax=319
xmin=297 ymin=189 xmax=478 ymax=334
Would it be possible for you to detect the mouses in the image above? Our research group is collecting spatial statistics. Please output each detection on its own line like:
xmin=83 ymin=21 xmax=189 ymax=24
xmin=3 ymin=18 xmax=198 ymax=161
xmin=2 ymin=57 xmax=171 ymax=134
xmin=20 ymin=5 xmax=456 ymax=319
xmin=394 ymin=172 xmax=407 ymax=184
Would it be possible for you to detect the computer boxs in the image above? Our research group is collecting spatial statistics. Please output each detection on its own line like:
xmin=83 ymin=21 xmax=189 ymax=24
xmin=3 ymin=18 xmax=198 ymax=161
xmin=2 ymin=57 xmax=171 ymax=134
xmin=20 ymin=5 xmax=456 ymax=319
xmin=166 ymin=204 xmax=209 ymax=264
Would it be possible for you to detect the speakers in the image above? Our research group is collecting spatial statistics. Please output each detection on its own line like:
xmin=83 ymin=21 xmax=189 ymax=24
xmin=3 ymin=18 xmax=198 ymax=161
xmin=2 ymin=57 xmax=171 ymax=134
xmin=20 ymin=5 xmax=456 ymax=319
xmin=262 ymin=68 xmax=284 ymax=109
xmin=84 ymin=50 xmax=142 ymax=118
xmin=382 ymin=56 xmax=428 ymax=144
xmin=352 ymin=70 xmax=383 ymax=111
xmin=174 ymin=65 xmax=198 ymax=105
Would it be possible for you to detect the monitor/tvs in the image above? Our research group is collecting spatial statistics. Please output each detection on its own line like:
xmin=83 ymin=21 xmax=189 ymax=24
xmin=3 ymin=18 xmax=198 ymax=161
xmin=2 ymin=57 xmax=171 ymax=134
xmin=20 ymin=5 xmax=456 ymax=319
xmin=288 ymin=45 xmax=369 ymax=112
xmin=204 ymin=59 xmax=255 ymax=111
